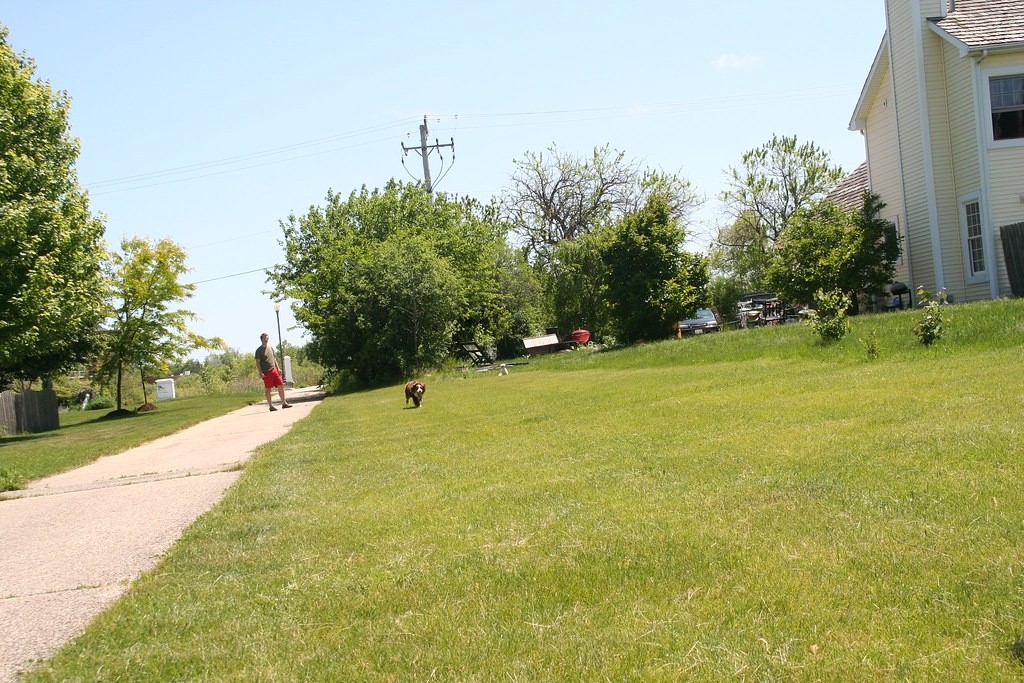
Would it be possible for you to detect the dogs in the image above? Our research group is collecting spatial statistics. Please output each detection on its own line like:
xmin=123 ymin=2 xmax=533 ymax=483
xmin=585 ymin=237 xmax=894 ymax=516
xmin=499 ymin=364 xmax=508 ymax=376
xmin=405 ymin=381 xmax=425 ymax=407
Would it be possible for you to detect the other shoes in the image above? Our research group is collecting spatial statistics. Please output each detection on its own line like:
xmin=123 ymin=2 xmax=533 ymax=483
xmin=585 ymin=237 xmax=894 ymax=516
xmin=281 ymin=403 xmax=292 ymax=408
xmin=269 ymin=406 xmax=277 ymax=411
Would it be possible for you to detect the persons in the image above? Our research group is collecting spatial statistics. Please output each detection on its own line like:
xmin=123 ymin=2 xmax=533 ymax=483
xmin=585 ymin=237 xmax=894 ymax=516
xmin=255 ymin=333 xmax=293 ymax=411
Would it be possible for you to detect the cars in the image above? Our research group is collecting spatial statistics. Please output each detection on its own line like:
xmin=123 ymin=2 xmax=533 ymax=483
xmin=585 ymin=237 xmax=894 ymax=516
xmin=679 ymin=307 xmax=720 ymax=338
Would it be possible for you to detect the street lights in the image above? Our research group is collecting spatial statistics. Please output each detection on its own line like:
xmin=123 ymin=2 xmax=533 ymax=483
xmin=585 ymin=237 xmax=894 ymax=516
xmin=275 ymin=301 xmax=286 ymax=384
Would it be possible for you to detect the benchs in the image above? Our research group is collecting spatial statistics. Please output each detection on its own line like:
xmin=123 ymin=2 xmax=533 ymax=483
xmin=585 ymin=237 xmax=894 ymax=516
xmin=522 ymin=334 xmax=576 ymax=354
